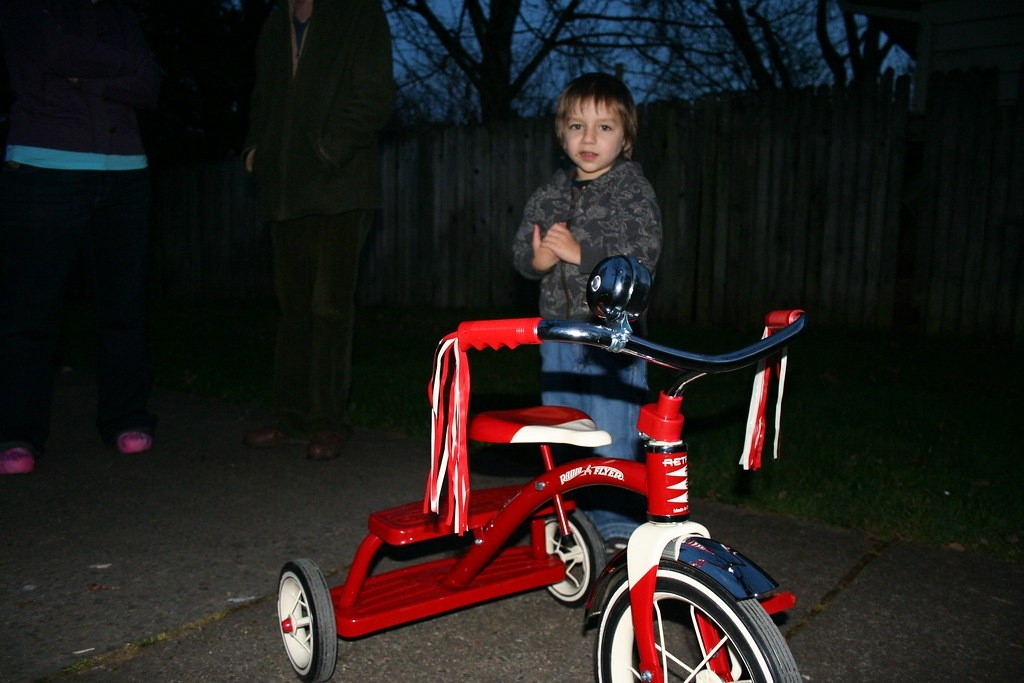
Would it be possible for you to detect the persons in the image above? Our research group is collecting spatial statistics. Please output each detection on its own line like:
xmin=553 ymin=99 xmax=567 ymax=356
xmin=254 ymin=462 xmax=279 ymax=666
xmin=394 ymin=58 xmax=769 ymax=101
xmin=0 ymin=1 xmax=160 ymax=474
xmin=512 ymin=72 xmax=671 ymax=620
xmin=239 ymin=0 xmax=394 ymax=460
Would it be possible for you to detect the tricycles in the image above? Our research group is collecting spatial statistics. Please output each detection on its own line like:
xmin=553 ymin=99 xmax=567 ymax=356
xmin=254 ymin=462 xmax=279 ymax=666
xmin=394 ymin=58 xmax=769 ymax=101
xmin=276 ymin=254 xmax=810 ymax=683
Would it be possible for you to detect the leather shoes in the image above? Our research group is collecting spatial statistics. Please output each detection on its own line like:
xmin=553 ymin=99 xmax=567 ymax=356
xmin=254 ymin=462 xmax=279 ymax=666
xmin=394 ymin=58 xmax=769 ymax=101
xmin=244 ymin=428 xmax=311 ymax=448
xmin=309 ymin=431 xmax=342 ymax=460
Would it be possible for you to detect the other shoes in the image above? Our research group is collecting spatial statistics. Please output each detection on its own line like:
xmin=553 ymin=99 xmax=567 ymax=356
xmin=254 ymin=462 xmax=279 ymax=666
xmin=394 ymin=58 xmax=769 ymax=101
xmin=604 ymin=537 xmax=629 ymax=560
xmin=117 ymin=431 xmax=152 ymax=454
xmin=0 ymin=448 xmax=35 ymax=475
